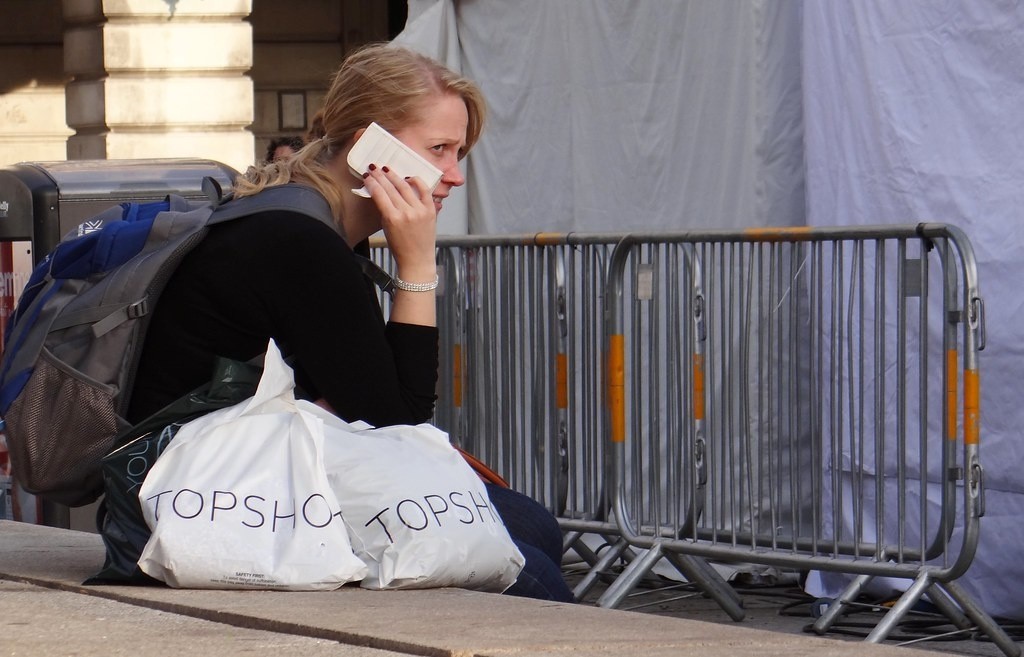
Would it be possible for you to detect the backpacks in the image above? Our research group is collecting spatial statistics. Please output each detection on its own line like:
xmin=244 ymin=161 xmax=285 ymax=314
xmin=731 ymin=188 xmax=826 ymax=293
xmin=0 ymin=176 xmax=349 ymax=508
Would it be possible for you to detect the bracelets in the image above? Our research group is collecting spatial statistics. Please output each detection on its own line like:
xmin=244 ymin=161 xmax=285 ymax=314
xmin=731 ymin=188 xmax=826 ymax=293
xmin=394 ymin=273 xmax=438 ymax=292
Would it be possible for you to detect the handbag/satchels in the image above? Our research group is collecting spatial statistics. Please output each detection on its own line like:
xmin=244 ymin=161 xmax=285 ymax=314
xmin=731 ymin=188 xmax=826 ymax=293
xmin=139 ymin=339 xmax=364 ymax=592
xmin=81 ymin=426 xmax=167 ymax=592
xmin=292 ymin=397 xmax=525 ymax=594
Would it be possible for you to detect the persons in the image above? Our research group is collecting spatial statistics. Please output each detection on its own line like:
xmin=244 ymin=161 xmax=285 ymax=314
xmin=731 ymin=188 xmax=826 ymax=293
xmin=85 ymin=49 xmax=577 ymax=603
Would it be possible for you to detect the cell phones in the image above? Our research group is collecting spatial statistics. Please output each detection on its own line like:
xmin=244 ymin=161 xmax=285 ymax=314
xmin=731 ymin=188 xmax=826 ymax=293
xmin=346 ymin=122 xmax=444 ymax=197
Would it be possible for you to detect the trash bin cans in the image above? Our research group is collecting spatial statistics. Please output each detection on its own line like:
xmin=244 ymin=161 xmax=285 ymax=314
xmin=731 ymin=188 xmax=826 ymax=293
xmin=7 ymin=158 xmax=242 ymax=269
xmin=0 ymin=170 xmax=35 ymax=349
